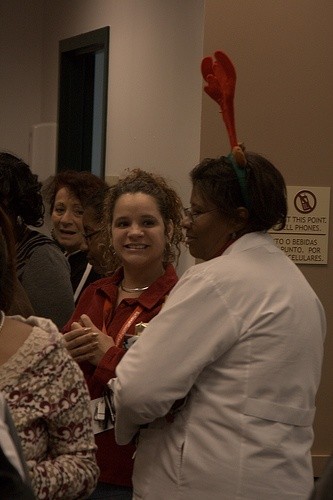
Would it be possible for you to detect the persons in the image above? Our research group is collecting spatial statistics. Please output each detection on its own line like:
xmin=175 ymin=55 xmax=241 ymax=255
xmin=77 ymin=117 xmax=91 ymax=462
xmin=108 ymin=152 xmax=327 ymax=500
xmin=0 ymin=153 xmax=110 ymax=500
xmin=61 ymin=167 xmax=186 ymax=500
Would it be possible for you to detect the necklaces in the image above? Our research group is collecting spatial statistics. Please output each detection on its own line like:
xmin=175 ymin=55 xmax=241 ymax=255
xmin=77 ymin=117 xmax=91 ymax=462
xmin=119 ymin=281 xmax=150 ymax=293
xmin=0 ymin=311 xmax=7 ymax=329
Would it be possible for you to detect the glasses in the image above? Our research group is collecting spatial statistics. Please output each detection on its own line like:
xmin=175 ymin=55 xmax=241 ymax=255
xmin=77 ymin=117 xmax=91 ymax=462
xmin=184 ymin=206 xmax=229 ymax=219
xmin=81 ymin=229 xmax=103 ymax=241
